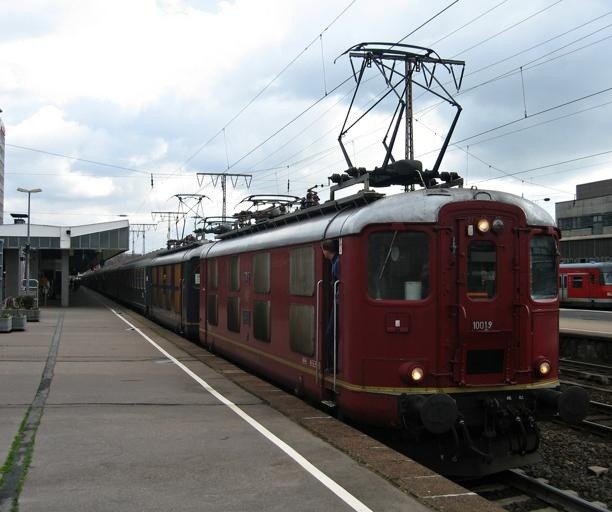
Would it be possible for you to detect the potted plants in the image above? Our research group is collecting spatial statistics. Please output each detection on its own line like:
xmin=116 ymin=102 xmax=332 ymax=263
xmin=0 ymin=293 xmax=41 ymax=333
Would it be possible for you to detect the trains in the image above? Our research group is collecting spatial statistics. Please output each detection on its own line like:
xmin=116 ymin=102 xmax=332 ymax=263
xmin=560 ymin=263 xmax=612 ymax=309
xmin=82 ymin=160 xmax=560 ymax=469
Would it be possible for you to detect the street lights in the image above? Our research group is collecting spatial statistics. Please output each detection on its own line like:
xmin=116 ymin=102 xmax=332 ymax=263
xmin=18 ymin=188 xmax=42 ymax=295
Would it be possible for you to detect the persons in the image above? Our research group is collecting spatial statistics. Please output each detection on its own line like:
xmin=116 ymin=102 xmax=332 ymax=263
xmin=320 ymin=240 xmax=340 ymax=375
